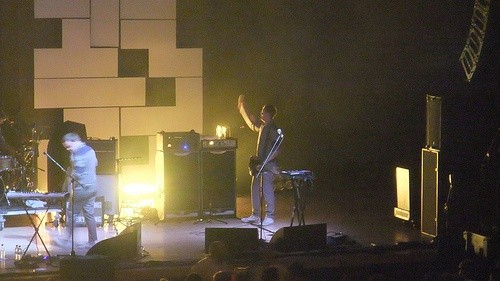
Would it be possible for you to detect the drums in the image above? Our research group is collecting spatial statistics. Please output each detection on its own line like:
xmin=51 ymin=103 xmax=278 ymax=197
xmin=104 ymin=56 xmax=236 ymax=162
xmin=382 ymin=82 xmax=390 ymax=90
xmin=0 ymin=156 xmax=17 ymax=172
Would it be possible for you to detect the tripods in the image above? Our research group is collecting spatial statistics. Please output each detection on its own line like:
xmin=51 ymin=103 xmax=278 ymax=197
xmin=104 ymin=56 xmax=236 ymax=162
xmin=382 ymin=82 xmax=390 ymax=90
xmin=194 ymin=200 xmax=228 ymax=225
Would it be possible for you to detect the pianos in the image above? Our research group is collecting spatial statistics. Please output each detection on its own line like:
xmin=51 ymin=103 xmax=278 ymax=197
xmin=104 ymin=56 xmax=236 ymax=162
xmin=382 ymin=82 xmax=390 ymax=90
xmin=7 ymin=191 xmax=69 ymax=202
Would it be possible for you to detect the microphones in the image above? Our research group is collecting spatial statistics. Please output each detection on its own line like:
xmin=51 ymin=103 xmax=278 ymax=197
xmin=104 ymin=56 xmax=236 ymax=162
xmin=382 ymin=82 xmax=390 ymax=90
xmin=277 ymin=129 xmax=282 ymax=136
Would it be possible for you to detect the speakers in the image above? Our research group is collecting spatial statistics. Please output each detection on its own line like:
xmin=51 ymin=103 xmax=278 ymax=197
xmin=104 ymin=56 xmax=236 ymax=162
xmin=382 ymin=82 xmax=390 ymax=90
xmin=426 ymin=90 xmax=494 ymax=151
xmin=65 ymin=196 xmax=104 ymax=227
xmin=204 ymin=228 xmax=259 ymax=255
xmin=86 ymin=139 xmax=118 ymax=175
xmin=269 ymin=223 xmax=327 ymax=253
xmin=163 ymin=151 xmax=200 ymax=220
xmin=59 ymin=222 xmax=142 ymax=281
xmin=200 ymin=150 xmax=237 ymax=218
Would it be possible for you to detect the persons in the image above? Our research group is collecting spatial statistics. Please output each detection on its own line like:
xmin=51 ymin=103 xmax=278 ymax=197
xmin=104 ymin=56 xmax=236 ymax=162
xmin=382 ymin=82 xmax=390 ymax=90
xmin=183 ymin=239 xmax=500 ymax=281
xmin=53 ymin=131 xmax=98 ymax=247
xmin=239 ymin=98 xmax=280 ymax=226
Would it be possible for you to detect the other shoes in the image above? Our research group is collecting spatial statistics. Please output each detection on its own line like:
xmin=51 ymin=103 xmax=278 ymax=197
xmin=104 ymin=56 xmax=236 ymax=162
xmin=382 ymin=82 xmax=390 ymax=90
xmin=241 ymin=215 xmax=260 ymax=223
xmin=259 ymin=217 xmax=273 ymax=226
xmin=54 ymin=237 xmax=69 ymax=248
xmin=78 ymin=242 xmax=94 ymax=249
